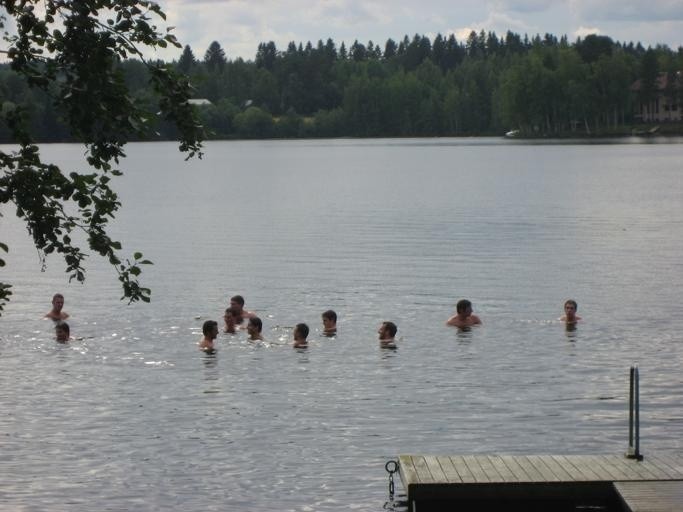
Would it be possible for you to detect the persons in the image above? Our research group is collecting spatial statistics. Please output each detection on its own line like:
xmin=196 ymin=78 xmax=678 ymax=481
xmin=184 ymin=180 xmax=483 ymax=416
xmin=321 ymin=309 xmax=336 ymax=332
xmin=377 ymin=321 xmax=396 ymax=340
xmin=221 ymin=295 xmax=256 ymax=318
xmin=223 ymin=307 xmax=238 ymax=334
xmin=559 ymin=300 xmax=582 ymax=322
xmin=43 ymin=293 xmax=69 ymax=321
xmin=198 ymin=320 xmax=219 ymax=349
xmin=53 ymin=322 xmax=72 ymax=343
xmin=246 ymin=317 xmax=263 ymax=340
xmin=445 ymin=299 xmax=480 ymax=328
xmin=291 ymin=323 xmax=308 ymax=345
